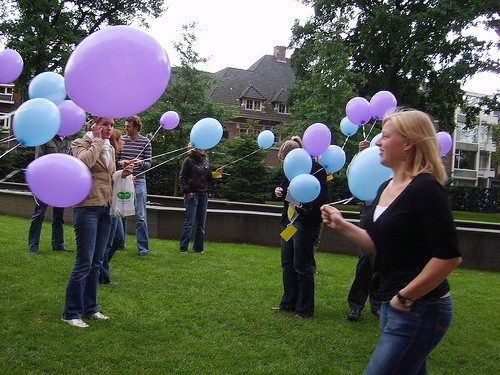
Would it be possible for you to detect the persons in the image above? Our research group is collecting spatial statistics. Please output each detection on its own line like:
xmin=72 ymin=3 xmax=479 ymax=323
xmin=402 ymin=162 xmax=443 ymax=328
xmin=271 ymin=136 xmax=327 ymax=319
xmin=179 ymin=141 xmax=223 ymax=254
xmin=61 ymin=116 xmax=133 ymax=329
xmin=320 ymin=110 xmax=462 ymax=375
xmin=116 ymin=115 xmax=152 ymax=256
xmin=348 ymin=202 xmax=382 ymax=320
xmin=100 ymin=128 xmax=125 ymax=285
xmin=28 ymin=134 xmax=72 ymax=253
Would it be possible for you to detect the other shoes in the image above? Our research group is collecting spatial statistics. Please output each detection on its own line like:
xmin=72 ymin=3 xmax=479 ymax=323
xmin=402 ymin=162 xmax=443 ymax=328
xmin=29 ymin=250 xmax=39 ymax=254
xmin=86 ymin=312 xmax=110 ymax=321
xmin=60 ymin=317 xmax=90 ymax=328
xmin=348 ymin=308 xmax=361 ymax=321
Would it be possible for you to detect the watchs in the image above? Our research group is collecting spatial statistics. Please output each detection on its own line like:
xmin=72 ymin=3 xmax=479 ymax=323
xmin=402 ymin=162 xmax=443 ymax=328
xmin=398 ymin=293 xmax=414 ymax=307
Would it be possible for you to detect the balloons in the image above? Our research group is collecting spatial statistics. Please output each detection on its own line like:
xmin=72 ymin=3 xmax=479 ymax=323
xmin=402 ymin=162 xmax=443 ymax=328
xmin=284 ymin=148 xmax=312 ymax=181
xmin=190 ymin=118 xmax=223 ymax=149
xmin=258 ymin=130 xmax=274 ymax=150
xmin=160 ymin=111 xmax=179 ymax=130
xmin=24 ymin=153 xmax=92 ymax=208
xmin=318 ymin=145 xmax=345 ymax=173
xmin=12 ymin=71 xmax=86 ymax=146
xmin=0 ymin=49 xmax=23 ymax=84
xmin=289 ymin=173 xmax=321 ymax=202
xmin=303 ymin=123 xmax=331 ymax=157
xmin=64 ymin=25 xmax=170 ymax=118
xmin=340 ymin=91 xmax=452 ymax=203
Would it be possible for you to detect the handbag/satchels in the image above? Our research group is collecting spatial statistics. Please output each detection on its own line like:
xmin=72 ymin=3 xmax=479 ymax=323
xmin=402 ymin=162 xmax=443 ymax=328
xmin=108 ymin=169 xmax=136 ymax=217
